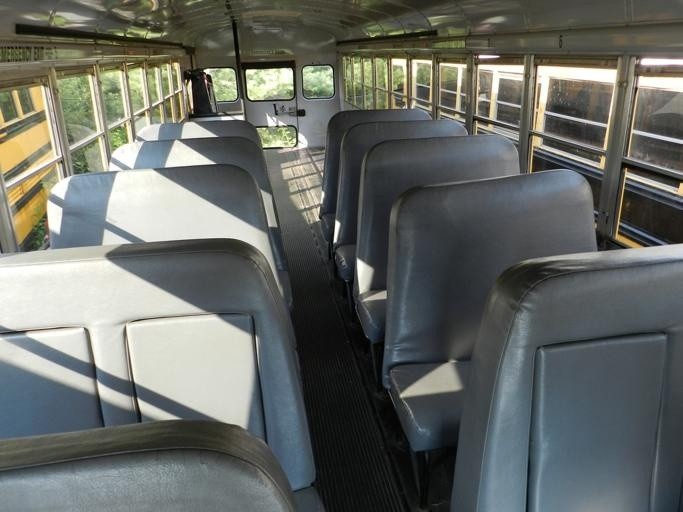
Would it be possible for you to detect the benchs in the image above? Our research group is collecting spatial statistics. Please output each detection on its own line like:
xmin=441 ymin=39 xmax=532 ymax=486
xmin=381 ymin=168 xmax=599 ymax=452
xmin=136 ymin=121 xmax=262 ymax=150
xmin=45 ymin=163 xmax=304 ymax=399
xmin=0 ymin=239 xmax=326 ymax=512
xmin=332 ymin=118 xmax=465 ymax=283
xmin=452 ymin=245 xmax=683 ymax=511
xmin=108 ymin=136 xmax=294 ymax=313
xmin=319 ymin=109 xmax=435 ymax=243
xmin=0 ymin=418 xmax=294 ymax=510
xmin=352 ymin=134 xmax=521 ymax=342
